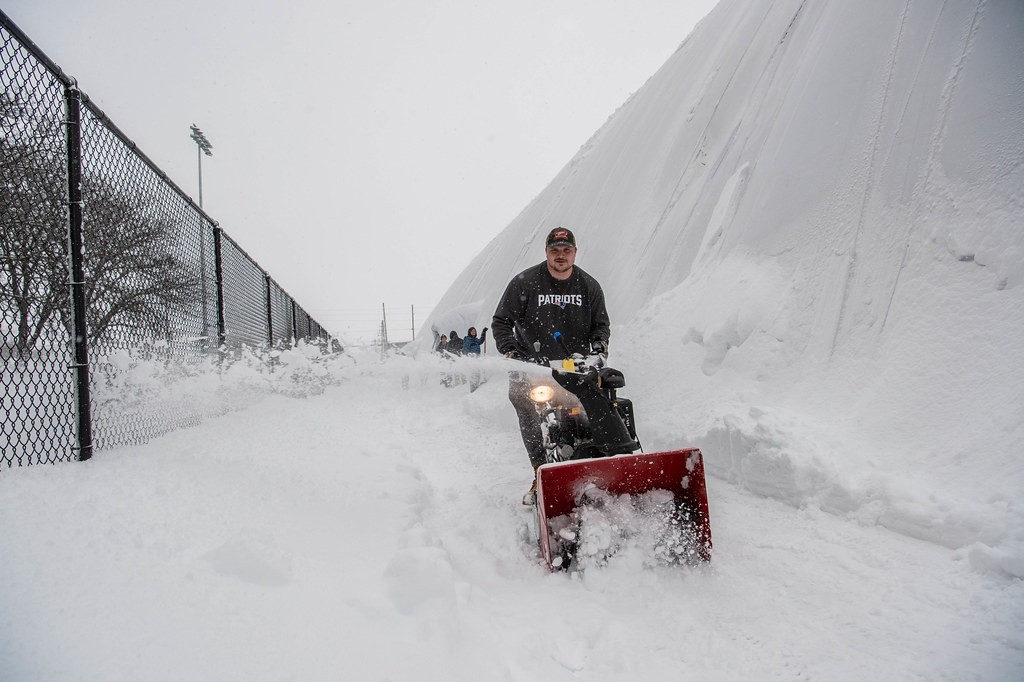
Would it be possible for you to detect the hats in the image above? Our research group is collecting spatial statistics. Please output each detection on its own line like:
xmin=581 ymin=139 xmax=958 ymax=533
xmin=546 ymin=227 xmax=576 ymax=248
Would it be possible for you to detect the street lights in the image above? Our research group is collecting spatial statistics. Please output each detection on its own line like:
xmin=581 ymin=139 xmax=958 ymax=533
xmin=190 ymin=119 xmax=212 ymax=349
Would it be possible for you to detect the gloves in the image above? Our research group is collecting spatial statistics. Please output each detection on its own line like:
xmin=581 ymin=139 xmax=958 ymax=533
xmin=483 ymin=328 xmax=488 ymax=332
xmin=504 ymin=347 xmax=523 ymax=358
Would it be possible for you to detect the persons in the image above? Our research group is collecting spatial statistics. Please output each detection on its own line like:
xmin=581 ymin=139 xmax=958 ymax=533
xmin=435 ymin=327 xmax=489 ymax=393
xmin=490 ymin=226 xmax=612 ymax=506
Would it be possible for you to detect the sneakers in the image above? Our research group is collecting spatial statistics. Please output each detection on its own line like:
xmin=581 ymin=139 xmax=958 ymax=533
xmin=523 ymin=465 xmax=540 ymax=505
